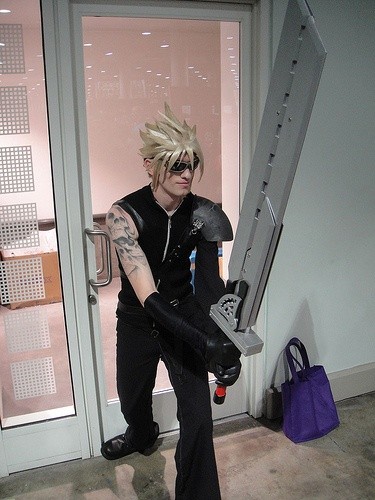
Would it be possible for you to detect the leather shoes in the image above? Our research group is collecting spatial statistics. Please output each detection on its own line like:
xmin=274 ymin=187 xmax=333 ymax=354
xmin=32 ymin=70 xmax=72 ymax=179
xmin=100 ymin=432 xmax=154 ymax=461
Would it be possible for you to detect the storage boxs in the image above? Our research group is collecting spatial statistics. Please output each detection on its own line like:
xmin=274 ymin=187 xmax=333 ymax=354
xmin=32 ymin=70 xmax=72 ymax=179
xmin=2 ymin=249 xmax=62 ymax=310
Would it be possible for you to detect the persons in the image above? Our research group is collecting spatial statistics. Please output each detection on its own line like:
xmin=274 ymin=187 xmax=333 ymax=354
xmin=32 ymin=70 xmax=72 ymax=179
xmin=99 ymin=102 xmax=243 ymax=500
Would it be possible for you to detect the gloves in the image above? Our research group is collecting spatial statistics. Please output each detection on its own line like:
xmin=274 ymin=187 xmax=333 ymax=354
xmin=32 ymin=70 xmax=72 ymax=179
xmin=205 ymin=352 xmax=242 ymax=386
xmin=200 ymin=335 xmax=241 ymax=370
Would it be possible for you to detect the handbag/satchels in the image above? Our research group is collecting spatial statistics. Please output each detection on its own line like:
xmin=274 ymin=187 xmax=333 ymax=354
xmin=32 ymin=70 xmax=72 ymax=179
xmin=281 ymin=337 xmax=341 ymax=444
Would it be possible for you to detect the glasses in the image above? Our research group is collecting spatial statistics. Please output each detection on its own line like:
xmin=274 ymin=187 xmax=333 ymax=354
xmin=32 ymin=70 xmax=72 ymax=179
xmin=156 ymin=158 xmax=199 ymax=173
